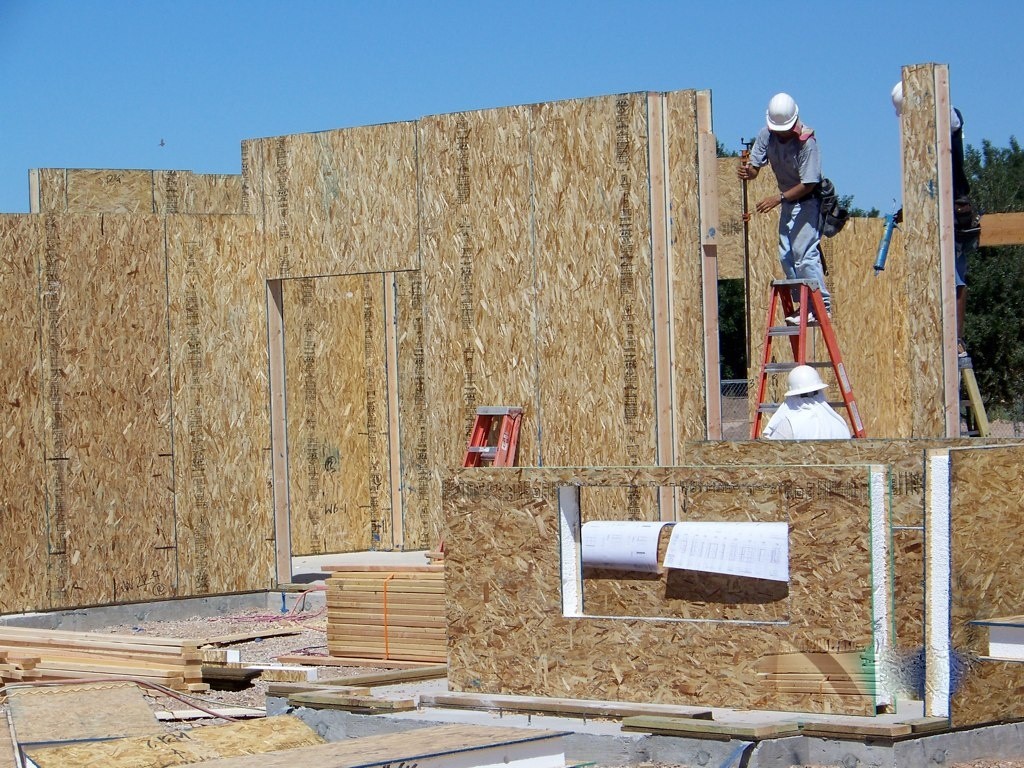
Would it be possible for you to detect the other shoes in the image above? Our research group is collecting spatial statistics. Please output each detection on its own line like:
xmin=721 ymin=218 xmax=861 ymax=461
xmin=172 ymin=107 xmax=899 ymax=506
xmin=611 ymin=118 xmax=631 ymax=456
xmin=957 ymin=339 xmax=967 ymax=358
xmin=786 ymin=309 xmax=807 ymax=323
xmin=790 ymin=311 xmax=830 ymax=325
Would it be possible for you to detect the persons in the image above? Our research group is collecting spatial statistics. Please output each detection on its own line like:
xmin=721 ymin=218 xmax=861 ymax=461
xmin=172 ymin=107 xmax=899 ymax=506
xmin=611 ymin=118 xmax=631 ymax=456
xmin=738 ymin=93 xmax=832 ymax=326
xmin=762 ymin=364 xmax=852 ymax=440
xmin=892 ymin=81 xmax=974 ymax=356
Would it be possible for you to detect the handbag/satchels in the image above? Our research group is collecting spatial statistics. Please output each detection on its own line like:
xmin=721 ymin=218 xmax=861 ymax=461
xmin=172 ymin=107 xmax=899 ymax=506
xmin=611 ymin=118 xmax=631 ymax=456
xmin=955 ymin=202 xmax=982 ymax=252
xmin=818 ymin=195 xmax=849 ymax=238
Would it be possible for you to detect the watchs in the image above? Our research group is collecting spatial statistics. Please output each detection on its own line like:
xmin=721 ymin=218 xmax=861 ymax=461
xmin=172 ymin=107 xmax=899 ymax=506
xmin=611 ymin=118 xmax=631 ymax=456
xmin=780 ymin=193 xmax=786 ymax=203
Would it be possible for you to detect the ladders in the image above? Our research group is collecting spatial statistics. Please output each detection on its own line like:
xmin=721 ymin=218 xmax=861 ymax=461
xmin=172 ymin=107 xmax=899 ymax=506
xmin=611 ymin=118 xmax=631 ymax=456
xmin=748 ymin=279 xmax=866 ymax=442
xmin=435 ymin=405 xmax=523 ymax=560
xmin=958 ymin=356 xmax=990 ymax=438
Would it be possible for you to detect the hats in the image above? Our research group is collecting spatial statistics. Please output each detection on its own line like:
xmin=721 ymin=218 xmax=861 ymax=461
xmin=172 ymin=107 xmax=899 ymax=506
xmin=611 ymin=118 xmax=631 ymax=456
xmin=766 ymin=93 xmax=799 ymax=132
xmin=783 ymin=365 xmax=830 ymax=397
xmin=891 ymin=81 xmax=904 ymax=118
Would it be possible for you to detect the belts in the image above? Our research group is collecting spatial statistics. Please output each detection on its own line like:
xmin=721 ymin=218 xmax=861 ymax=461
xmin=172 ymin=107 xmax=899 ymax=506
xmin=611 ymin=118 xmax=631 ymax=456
xmin=793 ymin=193 xmax=816 ymax=202
